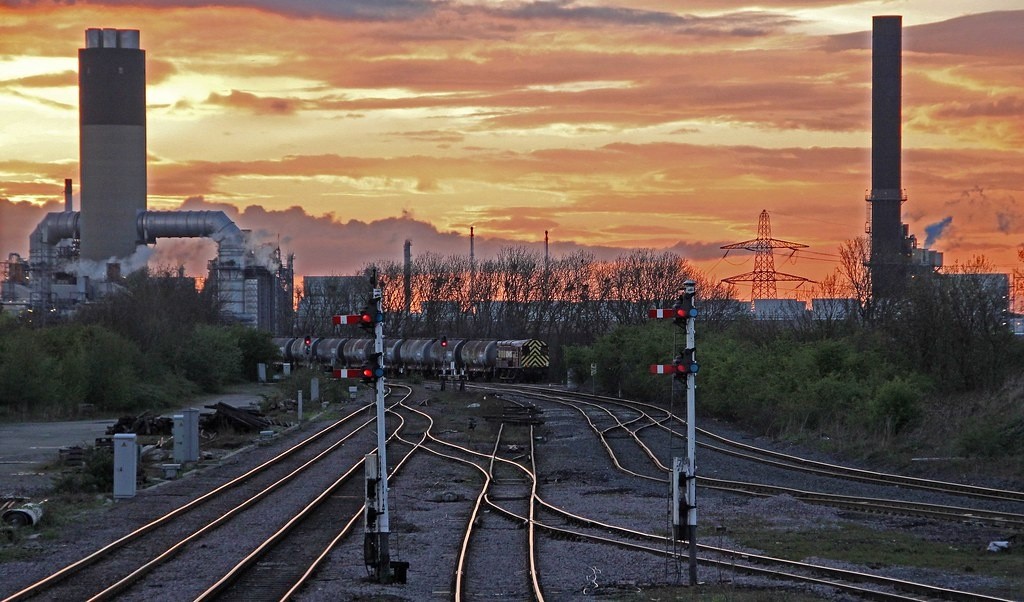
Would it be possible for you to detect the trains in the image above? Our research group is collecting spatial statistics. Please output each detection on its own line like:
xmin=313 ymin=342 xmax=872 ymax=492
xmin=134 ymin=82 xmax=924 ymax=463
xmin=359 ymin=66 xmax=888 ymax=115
xmin=272 ymin=337 xmax=551 ymax=384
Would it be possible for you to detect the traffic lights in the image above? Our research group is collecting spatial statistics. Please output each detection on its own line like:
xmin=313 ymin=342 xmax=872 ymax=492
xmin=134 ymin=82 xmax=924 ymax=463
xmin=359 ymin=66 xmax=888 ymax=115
xmin=646 ymin=292 xmax=700 ymax=377
xmin=439 ymin=336 xmax=449 ymax=351
xmin=303 ymin=333 xmax=314 ymax=351
xmin=331 ymin=299 xmax=386 ymax=381
